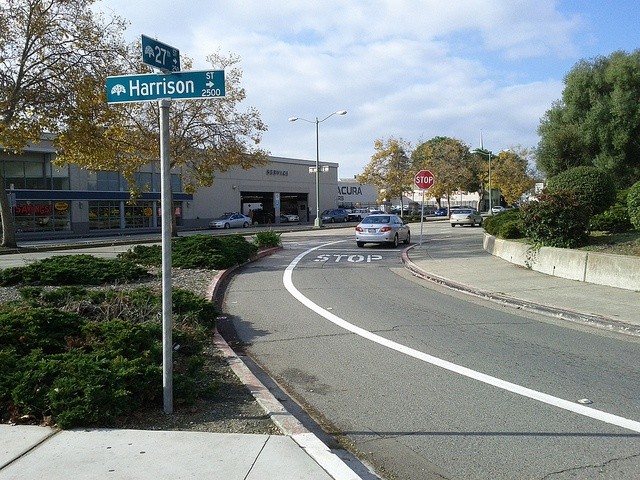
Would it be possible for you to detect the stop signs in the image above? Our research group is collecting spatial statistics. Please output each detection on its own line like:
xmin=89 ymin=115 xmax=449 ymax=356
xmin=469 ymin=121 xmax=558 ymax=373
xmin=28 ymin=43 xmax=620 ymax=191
xmin=414 ymin=170 xmax=435 ymax=189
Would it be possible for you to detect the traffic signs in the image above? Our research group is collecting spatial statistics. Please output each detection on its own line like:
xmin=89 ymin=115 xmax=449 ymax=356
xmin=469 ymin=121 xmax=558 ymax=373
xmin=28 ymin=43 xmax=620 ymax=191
xmin=142 ymin=34 xmax=180 ymax=72
xmin=106 ymin=69 xmax=225 ymax=105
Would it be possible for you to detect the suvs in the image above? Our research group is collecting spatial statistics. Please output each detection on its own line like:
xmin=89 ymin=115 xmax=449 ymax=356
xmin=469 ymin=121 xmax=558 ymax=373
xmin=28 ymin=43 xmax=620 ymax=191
xmin=253 ymin=209 xmax=275 ymax=224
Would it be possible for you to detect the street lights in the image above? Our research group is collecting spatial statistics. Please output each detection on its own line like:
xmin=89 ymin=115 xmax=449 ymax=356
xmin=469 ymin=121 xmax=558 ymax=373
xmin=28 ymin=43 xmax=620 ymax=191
xmin=288 ymin=111 xmax=347 ymax=229
xmin=469 ymin=148 xmax=492 ymax=215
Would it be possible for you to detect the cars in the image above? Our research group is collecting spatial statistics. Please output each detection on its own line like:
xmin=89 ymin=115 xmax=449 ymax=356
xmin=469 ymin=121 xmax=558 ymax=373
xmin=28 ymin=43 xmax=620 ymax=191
xmin=450 ymin=209 xmax=483 ymax=227
xmin=492 ymin=206 xmax=505 ymax=213
xmin=356 ymin=214 xmax=410 ymax=247
xmin=36 ymin=215 xmax=69 ymax=226
xmin=435 ymin=210 xmax=447 ymax=216
xmin=280 ymin=212 xmax=299 ymax=221
xmin=209 ymin=214 xmax=252 ymax=229
xmin=348 ymin=209 xmax=368 ymax=222
xmin=320 ymin=208 xmax=348 ymax=223
xmin=388 ymin=209 xmax=408 ymax=214
